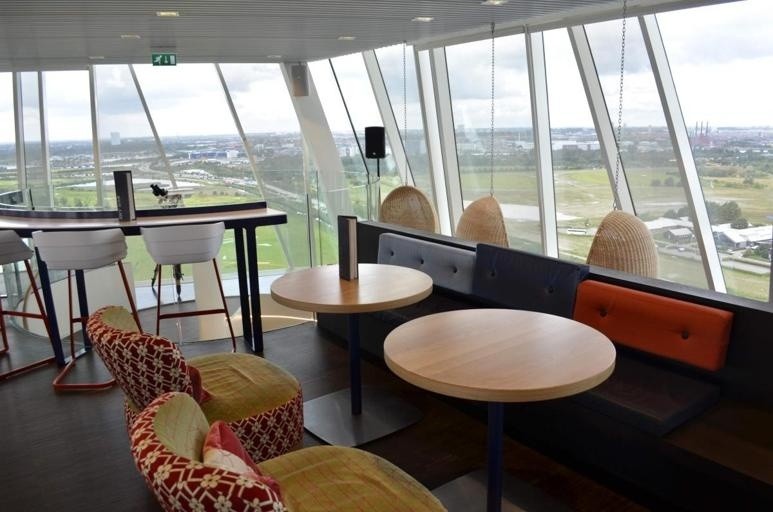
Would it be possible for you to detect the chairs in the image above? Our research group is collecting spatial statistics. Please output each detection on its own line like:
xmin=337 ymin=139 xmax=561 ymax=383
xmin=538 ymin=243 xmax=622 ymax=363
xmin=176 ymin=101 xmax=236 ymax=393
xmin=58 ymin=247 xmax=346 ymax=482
xmin=139 ymin=221 xmax=236 ymax=354
xmin=375 ymin=233 xmax=476 ymax=321
xmin=127 ymin=393 xmax=447 ymax=512
xmin=1 ymin=229 xmax=56 ymax=384
xmin=473 ymin=243 xmax=589 ymax=320
xmin=586 ymin=210 xmax=661 ymax=280
xmin=378 ymin=186 xmax=436 ymax=234
xmin=570 ymin=280 xmax=735 ymax=438
xmin=31 ymin=228 xmax=143 ymax=390
xmin=455 ymin=196 xmax=509 ymax=249
xmin=84 ymin=305 xmax=303 ymax=464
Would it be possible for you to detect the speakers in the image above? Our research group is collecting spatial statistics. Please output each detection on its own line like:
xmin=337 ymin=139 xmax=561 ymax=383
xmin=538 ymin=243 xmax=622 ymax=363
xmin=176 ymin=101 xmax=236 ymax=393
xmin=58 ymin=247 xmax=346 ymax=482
xmin=365 ymin=127 xmax=385 ymax=158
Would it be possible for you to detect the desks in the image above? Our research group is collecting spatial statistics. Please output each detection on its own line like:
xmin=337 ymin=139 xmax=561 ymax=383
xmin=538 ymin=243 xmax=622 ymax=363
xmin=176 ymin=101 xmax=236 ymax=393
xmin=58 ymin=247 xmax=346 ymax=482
xmin=0 ymin=200 xmax=289 ymax=358
xmin=272 ymin=263 xmax=433 ymax=448
xmin=383 ymin=307 xmax=617 ymax=512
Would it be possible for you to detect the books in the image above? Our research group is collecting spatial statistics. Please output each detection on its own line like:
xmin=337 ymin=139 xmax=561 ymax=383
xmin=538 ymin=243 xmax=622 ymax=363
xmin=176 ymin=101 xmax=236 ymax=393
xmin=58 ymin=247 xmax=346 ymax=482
xmin=337 ymin=214 xmax=359 ymax=281
xmin=111 ymin=169 xmax=137 ymax=224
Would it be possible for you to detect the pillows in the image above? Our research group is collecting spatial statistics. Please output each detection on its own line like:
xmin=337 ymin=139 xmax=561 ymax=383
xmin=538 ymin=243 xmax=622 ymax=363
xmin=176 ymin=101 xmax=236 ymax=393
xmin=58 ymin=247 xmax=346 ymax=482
xmin=188 ymin=364 xmax=217 ymax=403
xmin=202 ymin=421 xmax=286 ymax=508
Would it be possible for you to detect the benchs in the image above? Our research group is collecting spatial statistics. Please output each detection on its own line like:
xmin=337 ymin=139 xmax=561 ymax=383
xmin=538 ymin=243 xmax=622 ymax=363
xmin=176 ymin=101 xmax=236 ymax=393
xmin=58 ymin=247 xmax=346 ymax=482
xmin=662 ymin=398 xmax=772 ymax=511
